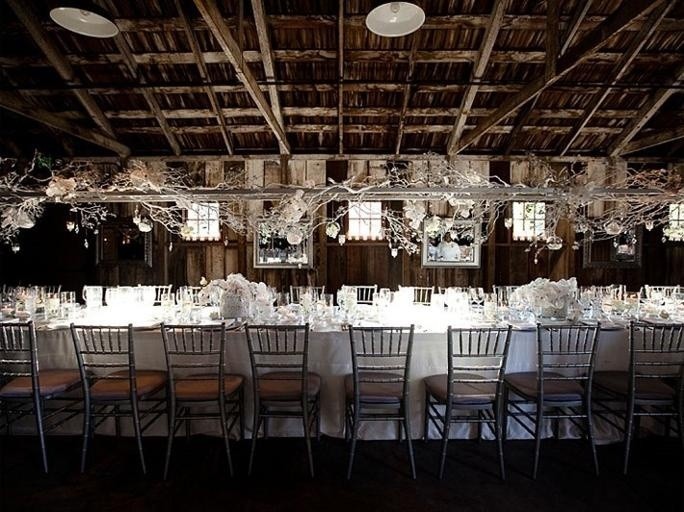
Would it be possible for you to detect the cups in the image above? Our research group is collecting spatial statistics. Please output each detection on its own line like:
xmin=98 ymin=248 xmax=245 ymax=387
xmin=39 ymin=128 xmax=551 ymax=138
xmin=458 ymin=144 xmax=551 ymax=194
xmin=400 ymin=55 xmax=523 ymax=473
xmin=1 ymin=286 xmax=194 ymax=326
xmin=320 ymin=286 xmax=513 ymax=326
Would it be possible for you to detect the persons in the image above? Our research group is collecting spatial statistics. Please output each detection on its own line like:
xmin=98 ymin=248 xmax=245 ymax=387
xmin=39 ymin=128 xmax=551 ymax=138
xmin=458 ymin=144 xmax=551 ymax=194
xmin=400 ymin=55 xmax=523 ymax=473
xmin=436 ymin=232 xmax=461 ymax=261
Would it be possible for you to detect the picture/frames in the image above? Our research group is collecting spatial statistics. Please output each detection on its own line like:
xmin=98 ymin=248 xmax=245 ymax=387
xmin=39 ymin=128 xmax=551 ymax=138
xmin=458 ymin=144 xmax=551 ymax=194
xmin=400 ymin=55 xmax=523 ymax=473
xmin=420 ymin=216 xmax=483 ymax=269
xmin=253 ymin=222 xmax=314 ymax=270
xmin=583 ymin=226 xmax=644 ymax=271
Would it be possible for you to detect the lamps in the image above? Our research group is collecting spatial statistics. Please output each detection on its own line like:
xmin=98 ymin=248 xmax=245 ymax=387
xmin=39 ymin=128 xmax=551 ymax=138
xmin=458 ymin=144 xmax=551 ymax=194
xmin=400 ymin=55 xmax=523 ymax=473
xmin=49 ymin=1 xmax=120 ymax=40
xmin=366 ymin=0 xmax=425 ymax=38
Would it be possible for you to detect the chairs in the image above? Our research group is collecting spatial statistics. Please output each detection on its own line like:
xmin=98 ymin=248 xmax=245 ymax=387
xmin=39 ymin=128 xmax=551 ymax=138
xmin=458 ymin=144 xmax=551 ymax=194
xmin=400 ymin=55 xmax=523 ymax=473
xmin=128 ymin=282 xmax=681 ymax=313
xmin=343 ymin=321 xmax=419 ymax=480
xmin=1 ymin=320 xmax=95 ymax=480
xmin=585 ymin=319 xmax=683 ymax=482
xmin=242 ymin=320 xmax=328 ymax=480
xmin=157 ymin=320 xmax=247 ymax=480
xmin=501 ymin=319 xmax=607 ymax=481
xmin=420 ymin=322 xmax=516 ymax=480
xmin=66 ymin=320 xmax=172 ymax=480
